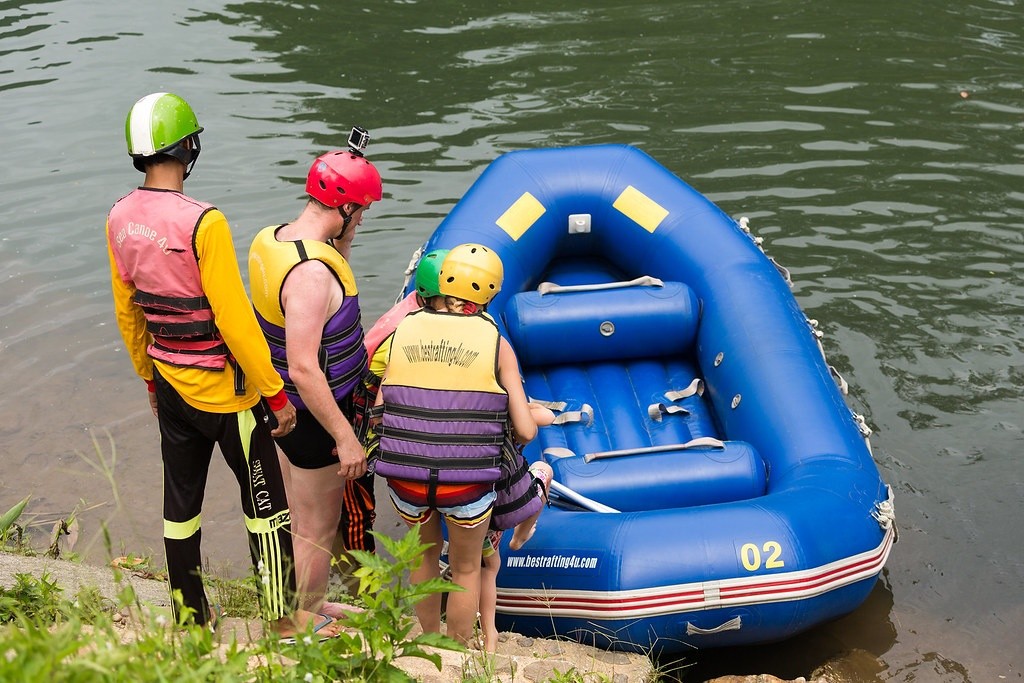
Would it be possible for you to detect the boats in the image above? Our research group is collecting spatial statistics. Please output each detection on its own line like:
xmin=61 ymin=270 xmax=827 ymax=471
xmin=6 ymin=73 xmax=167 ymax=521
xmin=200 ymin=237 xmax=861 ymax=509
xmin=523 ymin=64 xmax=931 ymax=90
xmin=399 ymin=142 xmax=896 ymax=655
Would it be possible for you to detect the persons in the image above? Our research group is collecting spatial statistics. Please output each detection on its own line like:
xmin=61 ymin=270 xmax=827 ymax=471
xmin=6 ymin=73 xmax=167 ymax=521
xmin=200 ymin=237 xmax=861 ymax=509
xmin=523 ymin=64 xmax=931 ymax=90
xmin=105 ymin=92 xmax=346 ymax=646
xmin=248 ymin=149 xmax=383 ymax=620
xmin=342 ymin=243 xmax=556 ymax=654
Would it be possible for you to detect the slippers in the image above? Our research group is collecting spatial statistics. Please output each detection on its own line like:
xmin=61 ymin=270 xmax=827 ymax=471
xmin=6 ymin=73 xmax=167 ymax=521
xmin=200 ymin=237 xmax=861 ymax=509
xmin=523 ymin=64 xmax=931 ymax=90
xmin=190 ymin=606 xmax=216 ymax=642
xmin=271 ymin=612 xmax=345 ymax=645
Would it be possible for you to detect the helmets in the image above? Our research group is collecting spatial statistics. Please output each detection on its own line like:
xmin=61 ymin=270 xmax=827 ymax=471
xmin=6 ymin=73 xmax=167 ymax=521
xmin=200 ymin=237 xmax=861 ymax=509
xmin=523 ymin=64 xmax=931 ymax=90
xmin=305 ymin=151 xmax=382 ymax=209
xmin=439 ymin=243 xmax=504 ymax=305
xmin=414 ymin=250 xmax=448 ymax=298
xmin=126 ymin=93 xmax=204 ymax=157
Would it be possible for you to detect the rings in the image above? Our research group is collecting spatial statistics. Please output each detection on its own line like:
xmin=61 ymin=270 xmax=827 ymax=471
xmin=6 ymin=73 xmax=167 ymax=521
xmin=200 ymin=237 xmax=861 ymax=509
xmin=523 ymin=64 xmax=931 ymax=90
xmin=290 ymin=423 xmax=296 ymax=429
